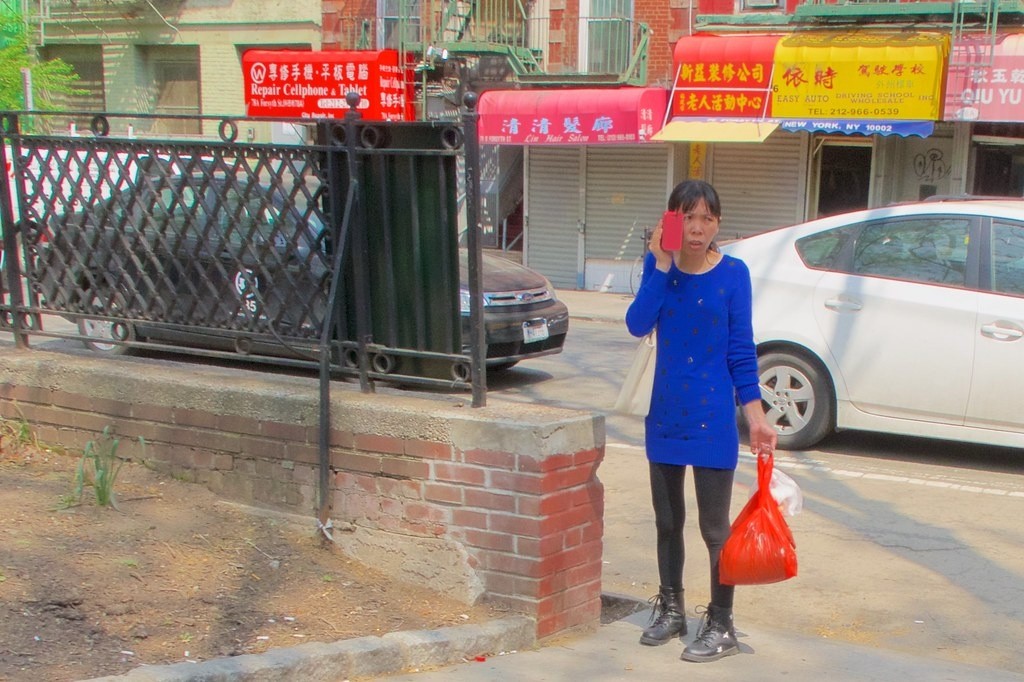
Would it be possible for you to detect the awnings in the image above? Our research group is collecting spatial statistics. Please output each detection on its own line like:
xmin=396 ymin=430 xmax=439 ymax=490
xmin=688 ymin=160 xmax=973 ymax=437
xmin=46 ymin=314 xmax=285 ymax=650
xmin=649 ymin=31 xmax=1024 ymax=142
xmin=476 ymin=88 xmax=673 ymax=145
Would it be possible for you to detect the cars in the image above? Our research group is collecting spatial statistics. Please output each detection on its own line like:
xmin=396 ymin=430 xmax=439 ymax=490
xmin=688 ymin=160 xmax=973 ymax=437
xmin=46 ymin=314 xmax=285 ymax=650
xmin=37 ymin=171 xmax=568 ymax=389
xmin=718 ymin=199 xmax=1024 ymax=451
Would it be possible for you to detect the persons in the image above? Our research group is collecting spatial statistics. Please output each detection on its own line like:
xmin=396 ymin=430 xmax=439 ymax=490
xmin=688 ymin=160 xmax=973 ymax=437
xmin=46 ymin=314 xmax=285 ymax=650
xmin=625 ymin=179 xmax=778 ymax=663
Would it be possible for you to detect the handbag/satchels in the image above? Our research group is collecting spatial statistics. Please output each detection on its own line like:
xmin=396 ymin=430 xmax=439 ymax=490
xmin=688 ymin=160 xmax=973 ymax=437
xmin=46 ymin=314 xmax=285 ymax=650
xmin=718 ymin=450 xmax=798 ymax=585
xmin=747 ymin=467 xmax=803 ymax=521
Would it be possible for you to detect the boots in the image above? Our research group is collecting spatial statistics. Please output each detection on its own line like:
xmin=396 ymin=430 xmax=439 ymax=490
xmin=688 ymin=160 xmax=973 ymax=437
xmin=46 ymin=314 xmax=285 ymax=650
xmin=681 ymin=602 xmax=740 ymax=662
xmin=639 ymin=585 xmax=688 ymax=646
